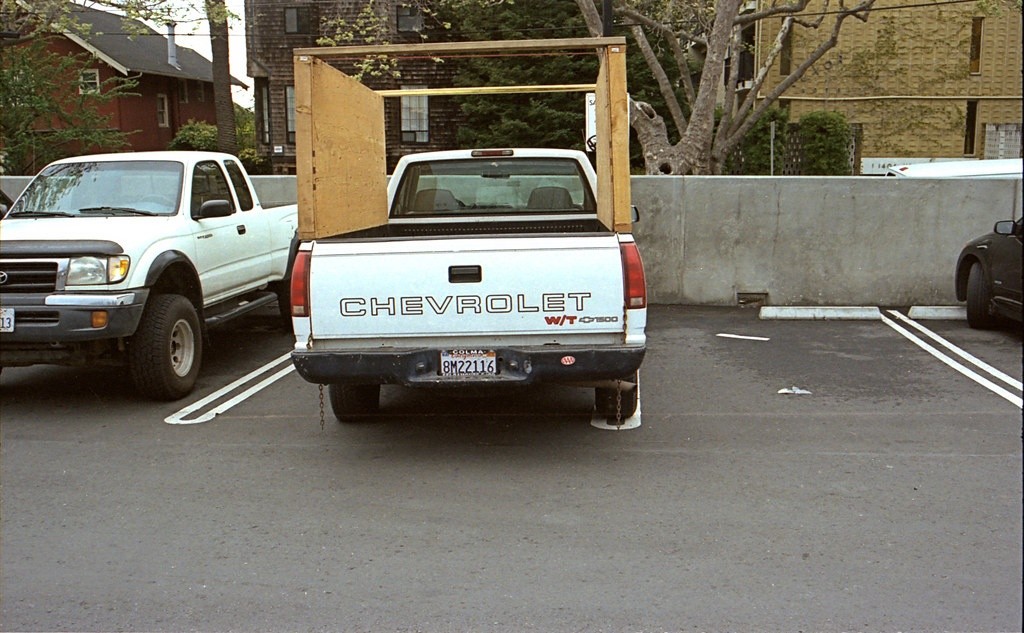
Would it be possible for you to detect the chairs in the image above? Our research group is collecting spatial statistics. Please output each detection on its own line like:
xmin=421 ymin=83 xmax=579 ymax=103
xmin=412 ymin=187 xmax=462 ymax=211
xmin=525 ymin=186 xmax=578 ymax=209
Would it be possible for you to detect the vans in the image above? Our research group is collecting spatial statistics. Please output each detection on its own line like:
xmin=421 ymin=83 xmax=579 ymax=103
xmin=883 ymin=157 xmax=1023 ymax=179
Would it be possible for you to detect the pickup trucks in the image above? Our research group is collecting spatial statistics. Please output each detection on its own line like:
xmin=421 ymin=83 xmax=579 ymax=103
xmin=289 ymin=147 xmax=649 ymax=421
xmin=0 ymin=150 xmax=298 ymax=404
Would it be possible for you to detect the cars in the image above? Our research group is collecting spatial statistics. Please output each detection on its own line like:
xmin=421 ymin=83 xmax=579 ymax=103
xmin=954 ymin=216 xmax=1024 ymax=330
